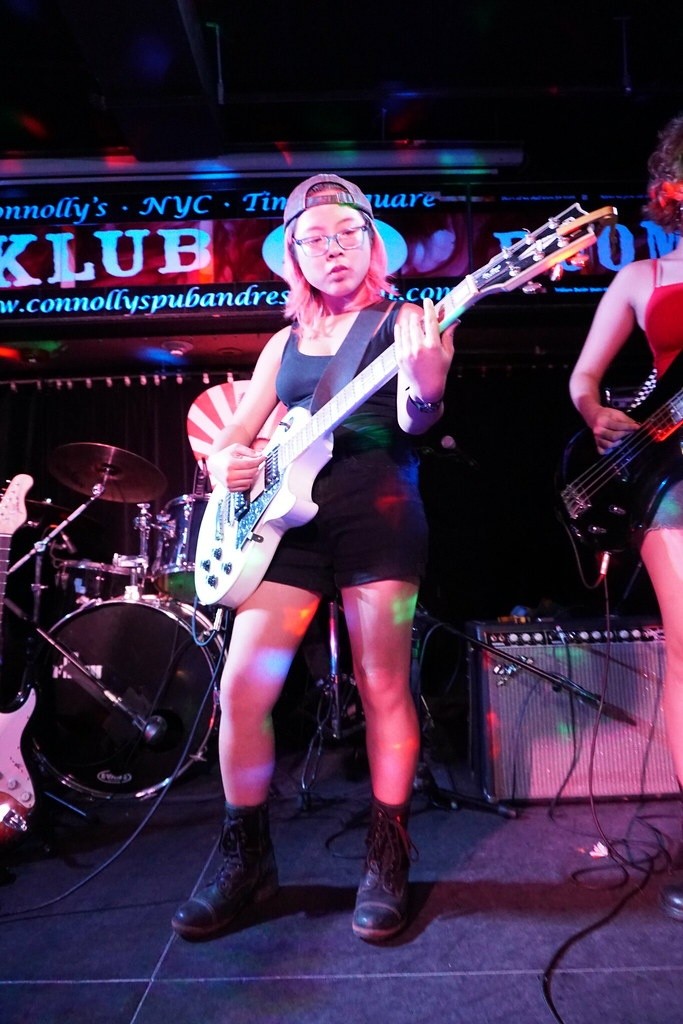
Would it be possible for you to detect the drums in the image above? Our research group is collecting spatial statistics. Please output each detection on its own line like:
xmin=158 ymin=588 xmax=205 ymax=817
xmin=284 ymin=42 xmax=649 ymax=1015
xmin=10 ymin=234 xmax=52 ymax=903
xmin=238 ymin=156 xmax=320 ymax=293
xmin=20 ymin=586 xmax=231 ymax=807
xmin=152 ymin=492 xmax=212 ymax=605
xmin=52 ymin=561 xmax=145 ymax=619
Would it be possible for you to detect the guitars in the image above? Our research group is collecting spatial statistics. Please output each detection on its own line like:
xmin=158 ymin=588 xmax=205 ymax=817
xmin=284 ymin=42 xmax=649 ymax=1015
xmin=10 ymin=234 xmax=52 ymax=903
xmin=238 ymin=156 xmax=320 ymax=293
xmin=0 ymin=471 xmax=38 ymax=846
xmin=550 ymin=347 xmax=683 ymax=590
xmin=190 ymin=199 xmax=619 ymax=610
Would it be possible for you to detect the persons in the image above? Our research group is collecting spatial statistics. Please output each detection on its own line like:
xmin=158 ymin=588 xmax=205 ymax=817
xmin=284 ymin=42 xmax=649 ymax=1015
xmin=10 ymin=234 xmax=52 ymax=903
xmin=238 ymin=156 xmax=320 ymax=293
xmin=170 ymin=173 xmax=461 ymax=942
xmin=569 ymin=114 xmax=683 ymax=923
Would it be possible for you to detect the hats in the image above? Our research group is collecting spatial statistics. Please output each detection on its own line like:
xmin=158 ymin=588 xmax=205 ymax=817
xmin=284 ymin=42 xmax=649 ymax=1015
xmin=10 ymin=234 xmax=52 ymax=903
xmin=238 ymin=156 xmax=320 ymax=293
xmin=283 ymin=173 xmax=373 ymax=230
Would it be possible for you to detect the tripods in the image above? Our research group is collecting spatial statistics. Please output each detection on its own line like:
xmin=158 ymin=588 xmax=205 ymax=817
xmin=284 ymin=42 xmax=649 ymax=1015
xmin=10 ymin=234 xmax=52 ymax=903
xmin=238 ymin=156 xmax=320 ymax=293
xmin=282 ymin=594 xmax=368 ymax=815
xmin=343 ymin=607 xmax=604 ymax=830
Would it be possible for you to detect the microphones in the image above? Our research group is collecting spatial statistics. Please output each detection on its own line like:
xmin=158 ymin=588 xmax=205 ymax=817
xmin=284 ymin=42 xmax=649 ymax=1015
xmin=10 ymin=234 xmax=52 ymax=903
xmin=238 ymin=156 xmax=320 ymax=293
xmin=60 ymin=531 xmax=77 ymax=555
xmin=141 ymin=716 xmax=167 ymax=746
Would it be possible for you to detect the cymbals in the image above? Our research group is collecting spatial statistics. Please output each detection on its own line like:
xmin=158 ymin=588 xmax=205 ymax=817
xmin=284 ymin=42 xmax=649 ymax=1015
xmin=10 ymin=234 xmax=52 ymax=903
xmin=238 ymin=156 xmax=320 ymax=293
xmin=44 ymin=439 xmax=170 ymax=504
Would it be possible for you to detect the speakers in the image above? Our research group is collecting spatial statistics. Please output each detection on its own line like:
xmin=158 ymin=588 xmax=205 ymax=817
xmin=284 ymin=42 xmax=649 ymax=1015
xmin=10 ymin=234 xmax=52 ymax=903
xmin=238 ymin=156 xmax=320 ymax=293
xmin=463 ymin=619 xmax=683 ymax=808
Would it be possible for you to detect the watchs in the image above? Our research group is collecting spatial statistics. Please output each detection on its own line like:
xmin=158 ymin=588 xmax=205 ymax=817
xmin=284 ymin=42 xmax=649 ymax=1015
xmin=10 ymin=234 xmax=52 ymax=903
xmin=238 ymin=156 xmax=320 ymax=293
xmin=405 ymin=386 xmax=444 ymax=413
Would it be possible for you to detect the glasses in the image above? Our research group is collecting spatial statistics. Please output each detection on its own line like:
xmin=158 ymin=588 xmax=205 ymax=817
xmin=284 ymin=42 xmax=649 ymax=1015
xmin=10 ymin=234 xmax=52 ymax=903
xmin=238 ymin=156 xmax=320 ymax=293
xmin=291 ymin=221 xmax=371 ymax=258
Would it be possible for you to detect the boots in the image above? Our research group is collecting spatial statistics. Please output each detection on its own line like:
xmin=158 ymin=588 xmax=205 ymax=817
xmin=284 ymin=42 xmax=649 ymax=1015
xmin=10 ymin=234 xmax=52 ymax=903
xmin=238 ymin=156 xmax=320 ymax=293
xmin=172 ymin=797 xmax=280 ymax=942
xmin=352 ymin=796 xmax=420 ymax=941
